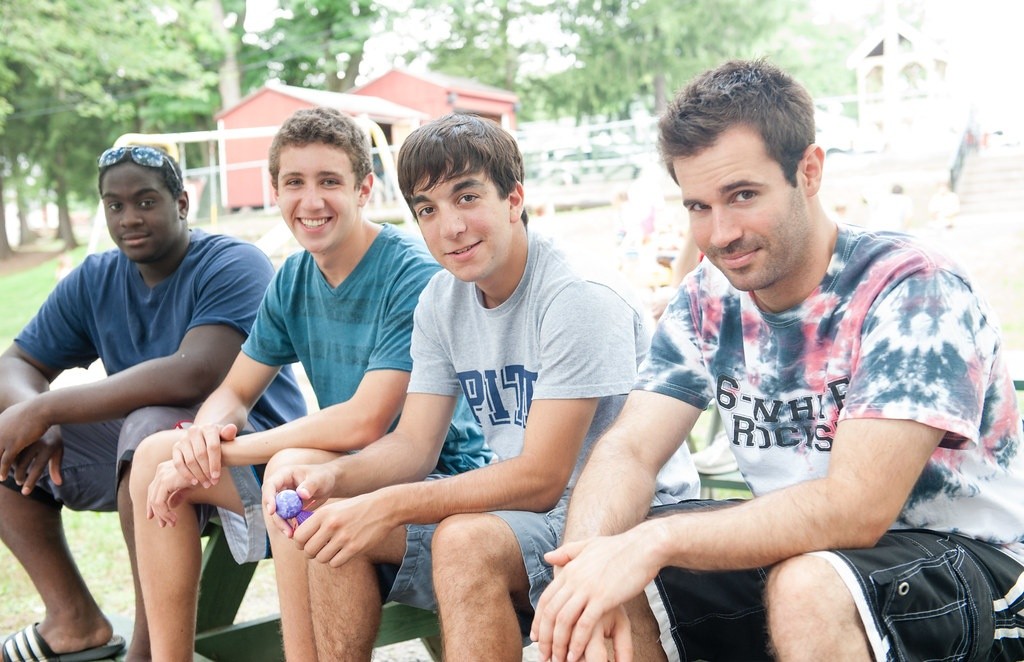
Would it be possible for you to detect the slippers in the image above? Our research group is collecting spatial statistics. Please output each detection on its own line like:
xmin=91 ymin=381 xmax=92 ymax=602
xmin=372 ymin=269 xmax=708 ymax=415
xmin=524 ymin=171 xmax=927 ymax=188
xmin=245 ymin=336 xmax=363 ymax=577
xmin=0 ymin=621 xmax=126 ymax=662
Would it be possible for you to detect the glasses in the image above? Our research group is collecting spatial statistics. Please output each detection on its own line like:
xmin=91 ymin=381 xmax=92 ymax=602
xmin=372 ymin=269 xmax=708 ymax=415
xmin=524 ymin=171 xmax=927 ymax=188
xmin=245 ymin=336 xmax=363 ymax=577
xmin=97 ymin=146 xmax=182 ymax=189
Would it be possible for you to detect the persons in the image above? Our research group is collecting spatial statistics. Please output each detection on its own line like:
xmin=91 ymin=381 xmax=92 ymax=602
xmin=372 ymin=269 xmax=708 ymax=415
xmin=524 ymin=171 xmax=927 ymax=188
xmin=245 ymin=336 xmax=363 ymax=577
xmin=670 ymin=221 xmax=737 ymax=475
xmin=265 ymin=111 xmax=702 ymax=662
xmin=129 ymin=106 xmax=496 ymax=662
xmin=530 ymin=58 xmax=1024 ymax=662
xmin=0 ymin=143 xmax=308 ymax=662
